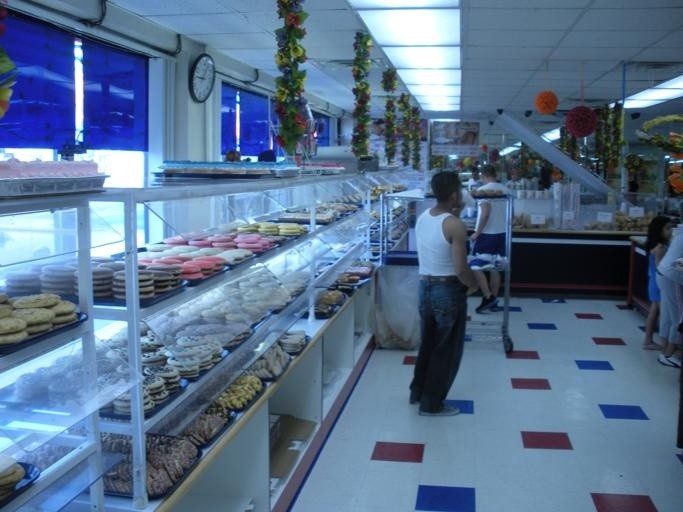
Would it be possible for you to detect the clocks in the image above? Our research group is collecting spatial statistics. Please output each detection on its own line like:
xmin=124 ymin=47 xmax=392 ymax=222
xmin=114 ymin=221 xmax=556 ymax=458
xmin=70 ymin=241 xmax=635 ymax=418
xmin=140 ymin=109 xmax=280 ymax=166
xmin=189 ymin=53 xmax=216 ymax=103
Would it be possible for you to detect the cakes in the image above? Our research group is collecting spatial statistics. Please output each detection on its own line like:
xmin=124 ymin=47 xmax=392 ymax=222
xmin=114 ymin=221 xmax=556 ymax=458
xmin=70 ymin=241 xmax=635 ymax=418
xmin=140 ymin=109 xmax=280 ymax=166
xmin=152 ymin=161 xmax=301 ymax=174
xmin=0 ymin=156 xmax=97 ymax=181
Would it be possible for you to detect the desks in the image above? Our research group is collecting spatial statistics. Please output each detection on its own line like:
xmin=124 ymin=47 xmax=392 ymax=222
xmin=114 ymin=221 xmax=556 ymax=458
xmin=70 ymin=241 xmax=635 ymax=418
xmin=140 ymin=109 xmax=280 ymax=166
xmin=628 ymin=234 xmax=654 ymax=318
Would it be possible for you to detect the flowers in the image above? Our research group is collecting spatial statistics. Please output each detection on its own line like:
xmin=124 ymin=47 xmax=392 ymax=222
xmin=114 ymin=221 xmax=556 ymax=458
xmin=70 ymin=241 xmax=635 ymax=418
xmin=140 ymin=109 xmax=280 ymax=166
xmin=272 ymin=0 xmax=422 ymax=174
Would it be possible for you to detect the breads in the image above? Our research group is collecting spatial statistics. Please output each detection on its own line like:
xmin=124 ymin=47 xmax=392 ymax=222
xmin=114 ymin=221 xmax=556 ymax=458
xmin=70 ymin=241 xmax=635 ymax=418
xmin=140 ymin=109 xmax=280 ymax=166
xmin=583 ymin=211 xmax=654 ymax=231
xmin=512 ymin=213 xmax=552 ymax=229
xmin=471 ymin=189 xmax=503 ymax=197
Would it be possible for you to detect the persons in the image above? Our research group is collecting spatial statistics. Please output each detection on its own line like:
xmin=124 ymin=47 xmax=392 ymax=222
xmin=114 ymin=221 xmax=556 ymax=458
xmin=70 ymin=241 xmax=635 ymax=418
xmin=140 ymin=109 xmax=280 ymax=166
xmin=642 ymin=215 xmax=673 ymax=351
xmin=466 ymin=164 xmax=511 ymax=314
xmin=654 ymin=202 xmax=683 ymax=369
xmin=407 ymin=171 xmax=480 ymax=418
xmin=540 ymin=159 xmax=560 ymax=191
xmin=467 ymin=167 xmax=480 ymax=218
xmin=505 ymin=168 xmax=519 ymax=186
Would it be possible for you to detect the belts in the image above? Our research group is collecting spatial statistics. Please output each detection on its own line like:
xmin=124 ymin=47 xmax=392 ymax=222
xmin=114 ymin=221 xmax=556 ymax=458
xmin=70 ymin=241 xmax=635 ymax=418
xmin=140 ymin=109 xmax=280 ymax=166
xmin=416 ymin=275 xmax=456 ymax=282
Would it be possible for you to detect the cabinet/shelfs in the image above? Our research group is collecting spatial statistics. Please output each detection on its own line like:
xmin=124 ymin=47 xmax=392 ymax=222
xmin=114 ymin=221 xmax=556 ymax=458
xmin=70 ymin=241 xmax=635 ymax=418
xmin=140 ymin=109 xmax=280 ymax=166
xmin=377 ymin=186 xmax=520 ymax=354
xmin=0 ymin=168 xmax=423 ymax=510
xmin=458 ymin=194 xmax=658 ymax=300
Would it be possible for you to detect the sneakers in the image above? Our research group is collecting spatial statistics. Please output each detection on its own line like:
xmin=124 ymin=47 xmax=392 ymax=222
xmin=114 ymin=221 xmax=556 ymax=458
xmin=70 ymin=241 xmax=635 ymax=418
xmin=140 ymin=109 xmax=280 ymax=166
xmin=643 ymin=342 xmax=680 ymax=367
xmin=476 ymin=294 xmax=500 ymax=313
xmin=411 ymin=396 xmax=460 ymax=417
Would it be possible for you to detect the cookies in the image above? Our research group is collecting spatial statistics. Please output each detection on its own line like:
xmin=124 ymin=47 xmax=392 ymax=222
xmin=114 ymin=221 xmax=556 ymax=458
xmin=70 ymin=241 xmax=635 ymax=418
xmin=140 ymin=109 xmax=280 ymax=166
xmin=0 ymin=199 xmax=359 ymax=500
